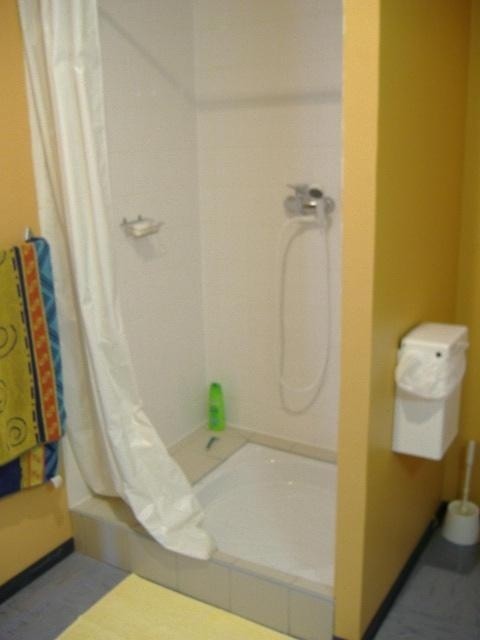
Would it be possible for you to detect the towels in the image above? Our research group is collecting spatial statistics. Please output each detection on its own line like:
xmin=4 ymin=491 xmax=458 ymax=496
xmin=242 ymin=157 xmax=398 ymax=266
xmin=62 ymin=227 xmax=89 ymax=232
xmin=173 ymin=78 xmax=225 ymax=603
xmin=1 ymin=237 xmax=67 ymax=498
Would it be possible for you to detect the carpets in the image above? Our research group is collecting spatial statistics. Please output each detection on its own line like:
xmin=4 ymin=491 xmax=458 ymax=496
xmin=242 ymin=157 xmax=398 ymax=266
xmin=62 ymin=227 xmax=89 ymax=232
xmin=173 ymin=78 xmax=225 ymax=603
xmin=54 ymin=571 xmax=300 ymax=639
